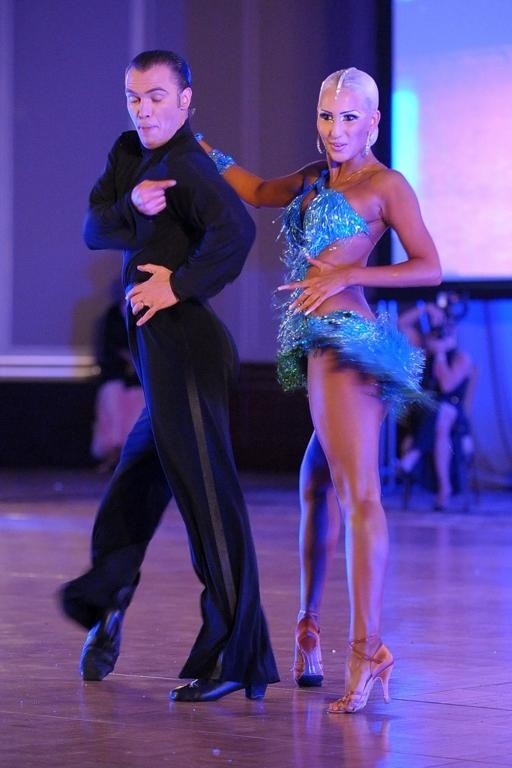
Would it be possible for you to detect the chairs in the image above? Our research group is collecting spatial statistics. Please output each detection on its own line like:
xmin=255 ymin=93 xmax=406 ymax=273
xmin=401 ymin=361 xmax=481 ymax=510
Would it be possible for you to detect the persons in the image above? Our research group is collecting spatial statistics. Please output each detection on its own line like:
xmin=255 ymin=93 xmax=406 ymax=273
xmin=394 ymin=298 xmax=474 ymax=515
xmin=189 ymin=65 xmax=443 ymax=714
xmin=55 ymin=50 xmax=285 ymax=703
xmin=88 ymin=277 xmax=148 ymax=479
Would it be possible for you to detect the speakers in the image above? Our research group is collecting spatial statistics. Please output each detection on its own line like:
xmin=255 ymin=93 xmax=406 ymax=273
xmin=0 ymin=377 xmax=102 ymax=469
xmin=228 ymin=361 xmax=314 ymax=474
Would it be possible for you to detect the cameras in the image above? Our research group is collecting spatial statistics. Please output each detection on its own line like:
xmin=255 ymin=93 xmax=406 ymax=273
xmin=428 ymin=323 xmax=448 ymax=339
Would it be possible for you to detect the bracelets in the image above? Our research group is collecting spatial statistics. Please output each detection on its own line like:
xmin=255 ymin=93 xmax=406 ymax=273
xmin=192 ymin=131 xmax=203 ymax=143
xmin=205 ymin=149 xmax=237 ymax=177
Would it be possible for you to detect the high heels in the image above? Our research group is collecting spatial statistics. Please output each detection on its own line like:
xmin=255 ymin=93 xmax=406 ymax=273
xmin=80 ymin=605 xmax=124 ymax=680
xmin=326 ymin=634 xmax=395 ymax=714
xmin=290 ymin=609 xmax=325 ymax=688
xmin=169 ymin=677 xmax=268 ymax=702
